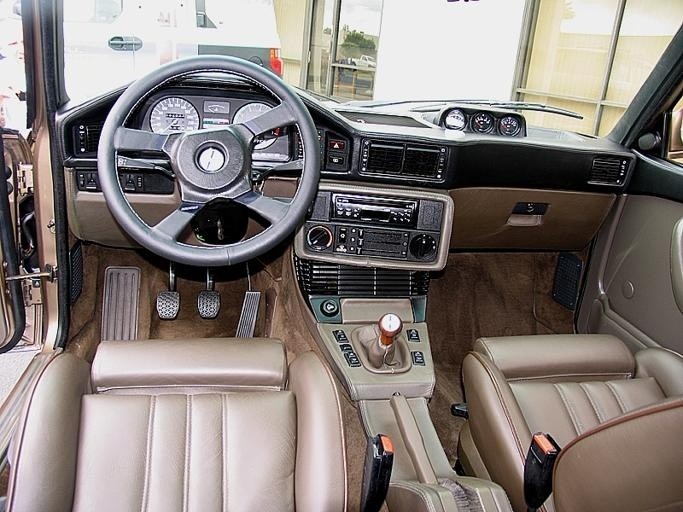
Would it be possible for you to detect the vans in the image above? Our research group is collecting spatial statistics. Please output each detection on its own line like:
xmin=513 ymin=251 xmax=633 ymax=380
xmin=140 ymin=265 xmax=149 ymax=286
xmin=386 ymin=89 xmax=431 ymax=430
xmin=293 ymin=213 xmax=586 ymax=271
xmin=0 ymin=0 xmax=283 ymax=143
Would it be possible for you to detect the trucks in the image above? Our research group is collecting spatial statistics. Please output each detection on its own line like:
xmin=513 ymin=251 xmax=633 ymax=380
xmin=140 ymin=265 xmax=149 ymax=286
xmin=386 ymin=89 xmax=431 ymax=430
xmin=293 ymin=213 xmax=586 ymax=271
xmin=348 ymin=53 xmax=376 ymax=71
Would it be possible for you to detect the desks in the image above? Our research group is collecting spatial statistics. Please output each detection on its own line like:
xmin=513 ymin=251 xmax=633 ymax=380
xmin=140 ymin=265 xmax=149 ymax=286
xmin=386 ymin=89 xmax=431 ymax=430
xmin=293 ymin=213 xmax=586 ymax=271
xmin=329 ymin=64 xmax=375 ymax=96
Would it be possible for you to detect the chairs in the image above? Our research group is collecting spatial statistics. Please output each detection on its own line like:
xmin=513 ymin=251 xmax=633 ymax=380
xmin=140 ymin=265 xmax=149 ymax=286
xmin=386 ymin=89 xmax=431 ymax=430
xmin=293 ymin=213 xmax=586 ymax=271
xmin=459 ymin=333 xmax=683 ymax=512
xmin=4 ymin=338 xmax=349 ymax=511
